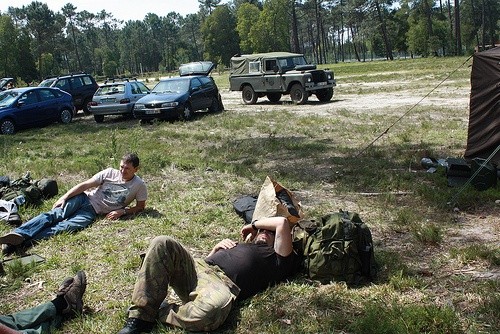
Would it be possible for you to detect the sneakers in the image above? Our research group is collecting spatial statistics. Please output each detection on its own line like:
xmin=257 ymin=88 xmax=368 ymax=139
xmin=116 ymin=317 xmax=151 ymax=334
xmin=56 ymin=270 xmax=87 ymax=317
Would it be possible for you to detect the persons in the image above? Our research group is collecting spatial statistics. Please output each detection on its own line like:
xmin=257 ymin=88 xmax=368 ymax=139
xmin=117 ymin=216 xmax=300 ymax=334
xmin=8 ymin=82 xmax=14 ymax=90
xmin=0 ymin=153 xmax=148 ymax=253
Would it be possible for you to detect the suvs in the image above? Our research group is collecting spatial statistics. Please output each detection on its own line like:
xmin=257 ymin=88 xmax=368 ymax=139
xmin=228 ymin=51 xmax=336 ymax=105
xmin=29 ymin=71 xmax=100 ymax=118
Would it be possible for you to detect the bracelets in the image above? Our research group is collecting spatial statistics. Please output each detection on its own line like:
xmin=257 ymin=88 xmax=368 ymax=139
xmin=252 ymin=220 xmax=258 ymax=230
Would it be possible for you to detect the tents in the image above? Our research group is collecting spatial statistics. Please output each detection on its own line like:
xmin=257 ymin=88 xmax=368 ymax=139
xmin=465 ymin=45 xmax=500 ymax=169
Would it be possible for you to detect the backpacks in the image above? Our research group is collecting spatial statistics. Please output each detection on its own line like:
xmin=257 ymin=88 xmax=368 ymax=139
xmin=304 ymin=212 xmax=374 ymax=287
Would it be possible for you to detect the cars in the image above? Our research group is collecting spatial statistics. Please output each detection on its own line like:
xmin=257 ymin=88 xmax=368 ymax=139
xmin=87 ymin=77 xmax=153 ymax=123
xmin=133 ymin=60 xmax=223 ymax=125
xmin=0 ymin=77 xmax=75 ymax=136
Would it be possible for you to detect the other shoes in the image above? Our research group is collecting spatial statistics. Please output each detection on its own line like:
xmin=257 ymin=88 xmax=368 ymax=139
xmin=0 ymin=234 xmax=24 ymax=245
xmin=1 ymin=243 xmax=11 ymax=253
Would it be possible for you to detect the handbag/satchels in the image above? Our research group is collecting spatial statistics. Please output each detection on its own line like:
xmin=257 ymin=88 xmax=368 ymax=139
xmin=30 ymin=179 xmax=59 ymax=200
